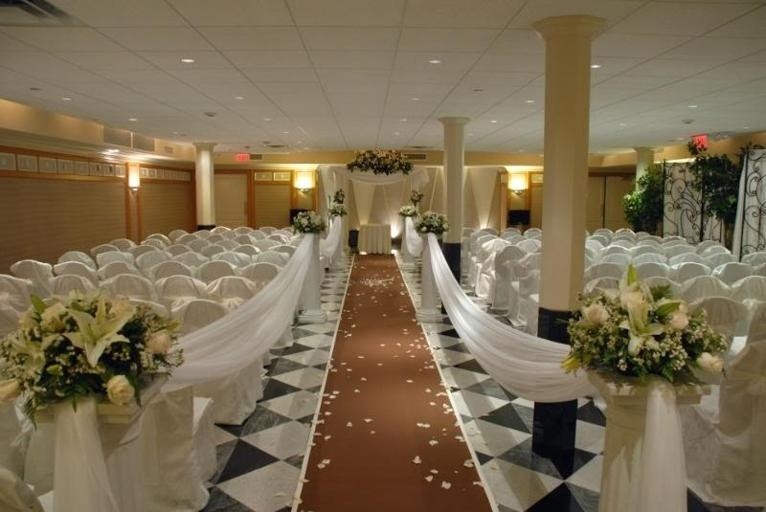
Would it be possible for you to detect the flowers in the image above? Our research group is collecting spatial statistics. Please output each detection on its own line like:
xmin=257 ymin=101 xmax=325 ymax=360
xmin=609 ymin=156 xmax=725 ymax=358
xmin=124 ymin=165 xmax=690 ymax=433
xmin=557 ymin=261 xmax=735 ymax=386
xmin=409 ymin=190 xmax=425 ymax=210
xmin=330 ymin=205 xmax=348 ymax=216
xmin=291 ymin=208 xmax=329 ymax=237
xmin=345 ymin=146 xmax=414 ymax=176
xmin=0 ymin=286 xmax=187 ymax=430
xmin=414 ymin=212 xmax=448 ymax=233
xmin=397 ymin=205 xmax=418 ymax=218
xmin=332 ymin=189 xmax=345 ymax=205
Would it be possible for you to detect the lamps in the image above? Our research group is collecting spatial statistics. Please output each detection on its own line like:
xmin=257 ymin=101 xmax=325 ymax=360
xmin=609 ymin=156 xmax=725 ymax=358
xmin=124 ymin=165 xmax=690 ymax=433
xmin=126 ymin=163 xmax=142 ymax=194
xmin=507 ymin=172 xmax=529 ymax=195
xmin=294 ymin=171 xmax=316 ymax=195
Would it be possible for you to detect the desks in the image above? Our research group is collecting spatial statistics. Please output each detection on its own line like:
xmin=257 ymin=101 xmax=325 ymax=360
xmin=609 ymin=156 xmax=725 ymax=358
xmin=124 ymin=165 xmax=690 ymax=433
xmin=357 ymin=222 xmax=392 ymax=256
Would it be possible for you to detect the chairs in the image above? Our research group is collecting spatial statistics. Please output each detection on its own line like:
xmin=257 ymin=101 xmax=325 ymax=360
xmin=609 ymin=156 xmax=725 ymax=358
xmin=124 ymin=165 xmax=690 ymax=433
xmin=643 ymin=275 xmax=684 ymax=295
xmin=1 ymin=224 xmax=315 ymax=300
xmin=685 ymin=296 xmax=742 ymax=328
xmin=580 ymin=275 xmax=617 ymax=291
xmin=171 ymin=299 xmax=269 ymax=428
xmin=475 ymin=234 xmax=524 ymax=311
xmin=208 ymin=277 xmax=279 ymax=370
xmin=731 ymin=275 xmax=766 ymax=303
xmin=0 ymin=303 xmax=22 ymax=338
xmin=146 ymin=385 xmax=217 ymax=511
xmin=460 ymin=227 xmax=766 ymax=285
xmin=678 ymin=340 xmax=766 ymax=508
xmin=502 ymin=252 xmax=540 ymax=329
xmin=729 ymin=301 xmax=766 ymax=344
xmin=130 ymin=298 xmax=168 ymax=321
xmin=680 ymin=274 xmax=728 ymax=296
